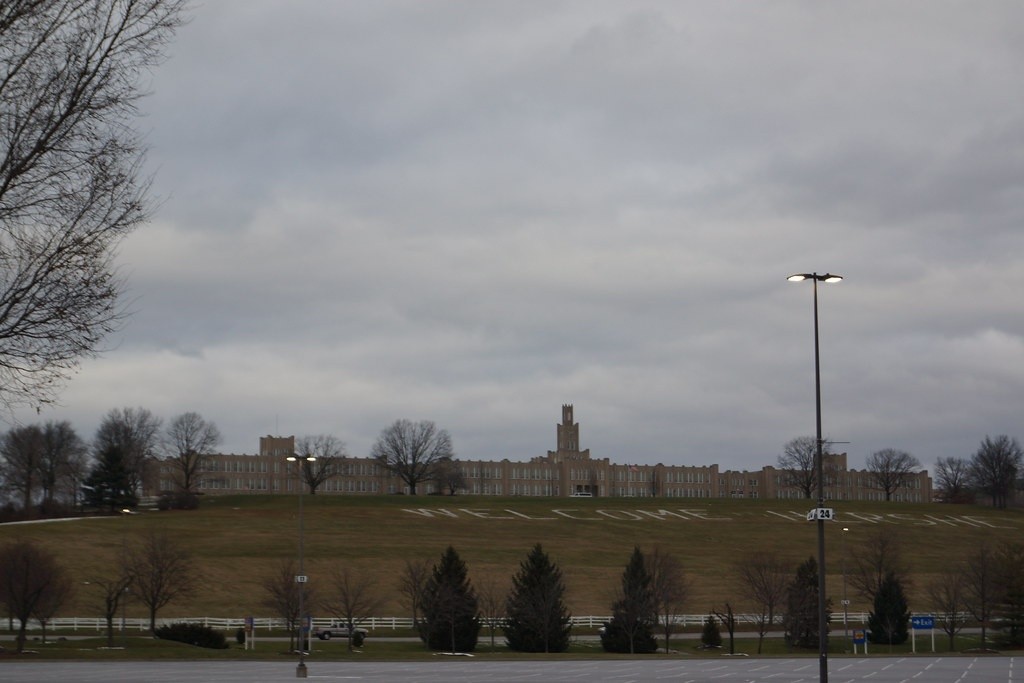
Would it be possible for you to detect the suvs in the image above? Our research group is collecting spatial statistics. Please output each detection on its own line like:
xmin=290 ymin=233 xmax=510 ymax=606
xmin=569 ymin=493 xmax=593 ymax=498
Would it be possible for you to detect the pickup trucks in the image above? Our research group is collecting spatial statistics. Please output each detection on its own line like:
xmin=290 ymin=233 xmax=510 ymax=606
xmin=313 ymin=622 xmax=369 ymax=641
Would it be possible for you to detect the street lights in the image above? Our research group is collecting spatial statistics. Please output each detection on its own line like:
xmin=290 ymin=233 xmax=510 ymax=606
xmin=787 ymin=270 xmax=845 ymax=682
xmin=287 ymin=453 xmax=317 ymax=678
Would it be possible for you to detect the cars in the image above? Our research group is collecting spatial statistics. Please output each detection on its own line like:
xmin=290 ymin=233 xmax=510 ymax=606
xmin=930 ymin=497 xmax=943 ymax=503
